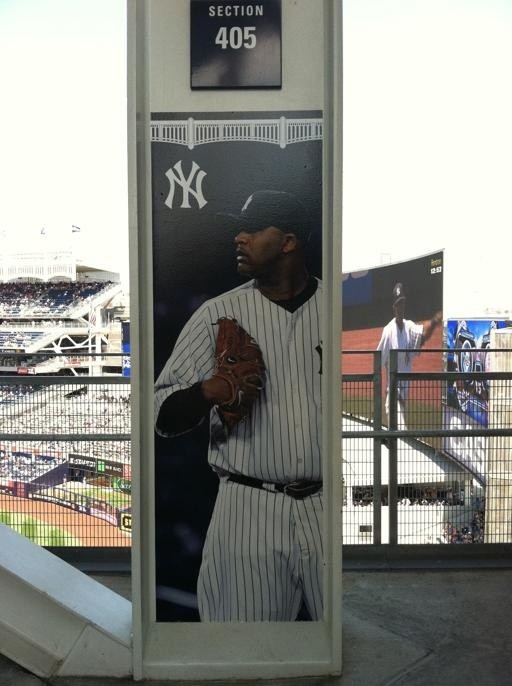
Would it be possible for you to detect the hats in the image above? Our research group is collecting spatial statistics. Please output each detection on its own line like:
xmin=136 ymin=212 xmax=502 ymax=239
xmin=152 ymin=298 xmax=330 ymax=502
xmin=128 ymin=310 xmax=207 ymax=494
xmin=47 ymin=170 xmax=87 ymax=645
xmin=216 ymin=190 xmax=310 ymax=244
xmin=393 ymin=283 xmax=406 ymax=305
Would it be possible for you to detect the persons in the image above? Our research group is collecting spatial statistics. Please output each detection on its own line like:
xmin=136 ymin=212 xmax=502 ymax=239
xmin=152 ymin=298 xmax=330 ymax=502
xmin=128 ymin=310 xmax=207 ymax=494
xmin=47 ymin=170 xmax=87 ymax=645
xmin=376 ymin=282 xmax=443 ymax=431
xmin=0 ymin=281 xmax=131 ymax=483
xmin=353 ymin=483 xmax=484 ymax=544
xmin=155 ymin=190 xmax=324 ymax=622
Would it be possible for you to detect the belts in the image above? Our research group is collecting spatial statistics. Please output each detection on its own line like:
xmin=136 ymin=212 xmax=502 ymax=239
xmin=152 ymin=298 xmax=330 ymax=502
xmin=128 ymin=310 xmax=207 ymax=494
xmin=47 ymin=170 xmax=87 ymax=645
xmin=227 ymin=474 xmax=322 ymax=499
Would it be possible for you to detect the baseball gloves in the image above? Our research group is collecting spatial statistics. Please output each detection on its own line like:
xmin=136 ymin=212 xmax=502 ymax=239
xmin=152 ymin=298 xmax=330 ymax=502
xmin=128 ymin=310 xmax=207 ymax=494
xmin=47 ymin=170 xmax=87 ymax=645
xmin=214 ymin=315 xmax=265 ymax=438
